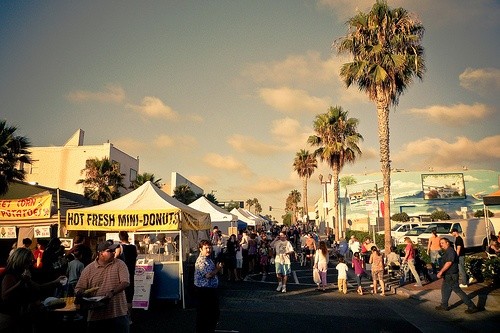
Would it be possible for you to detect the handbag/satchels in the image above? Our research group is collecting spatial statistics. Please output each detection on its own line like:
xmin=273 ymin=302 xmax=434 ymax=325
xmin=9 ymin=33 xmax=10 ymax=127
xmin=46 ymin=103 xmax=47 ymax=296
xmin=313 ymin=267 xmax=320 ymax=283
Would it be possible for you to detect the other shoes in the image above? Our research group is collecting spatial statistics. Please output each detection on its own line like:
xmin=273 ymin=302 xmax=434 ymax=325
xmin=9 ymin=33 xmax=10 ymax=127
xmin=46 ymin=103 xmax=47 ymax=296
xmin=315 ymin=289 xmax=320 ymax=292
xmin=371 ymin=290 xmax=376 ymax=294
xmin=276 ymin=285 xmax=282 ymax=291
xmin=459 ymin=283 xmax=468 ymax=288
xmin=469 ymin=277 xmax=477 ymax=283
xmin=321 ymin=290 xmax=326 ymax=292
xmin=435 ymin=305 xmax=449 ymax=311
xmin=380 ymin=294 xmax=385 ymax=296
xmin=464 ymin=308 xmax=478 ymax=314
xmin=282 ymin=287 xmax=286 ymax=292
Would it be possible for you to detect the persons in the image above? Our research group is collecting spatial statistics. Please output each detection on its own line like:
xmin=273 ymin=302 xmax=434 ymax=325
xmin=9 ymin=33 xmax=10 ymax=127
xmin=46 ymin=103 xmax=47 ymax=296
xmin=436 ymin=238 xmax=478 ymax=314
xmin=193 ymin=240 xmax=222 ymax=333
xmin=336 ymin=257 xmax=349 ymax=294
xmin=312 ymin=241 xmax=329 ymax=291
xmin=73 ymin=241 xmax=130 ymax=333
xmin=402 ymin=237 xmax=423 ymax=286
xmin=270 ymin=231 xmax=294 ymax=293
xmin=0 ymin=220 xmax=500 ymax=333
xmin=351 ymin=252 xmax=365 ymax=296
xmin=369 ymin=246 xmax=386 ymax=297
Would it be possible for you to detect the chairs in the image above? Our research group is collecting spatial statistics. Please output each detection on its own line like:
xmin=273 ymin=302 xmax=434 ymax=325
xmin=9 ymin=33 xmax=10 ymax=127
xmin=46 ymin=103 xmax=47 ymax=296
xmin=387 ymin=262 xmax=400 ymax=282
xmin=435 ymin=226 xmax=445 ymax=232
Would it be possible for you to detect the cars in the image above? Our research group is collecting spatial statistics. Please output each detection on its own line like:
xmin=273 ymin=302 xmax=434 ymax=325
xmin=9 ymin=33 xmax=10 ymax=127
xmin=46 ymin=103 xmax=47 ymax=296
xmin=398 ymin=225 xmax=429 ymax=249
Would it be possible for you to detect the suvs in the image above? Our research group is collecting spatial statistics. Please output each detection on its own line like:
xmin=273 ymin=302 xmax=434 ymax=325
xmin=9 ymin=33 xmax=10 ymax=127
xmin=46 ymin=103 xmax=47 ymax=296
xmin=376 ymin=222 xmax=434 ymax=246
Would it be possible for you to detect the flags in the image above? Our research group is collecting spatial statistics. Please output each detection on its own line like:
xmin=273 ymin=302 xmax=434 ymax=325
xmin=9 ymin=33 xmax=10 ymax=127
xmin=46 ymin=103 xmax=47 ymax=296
xmin=0 ymin=191 xmax=56 ymax=220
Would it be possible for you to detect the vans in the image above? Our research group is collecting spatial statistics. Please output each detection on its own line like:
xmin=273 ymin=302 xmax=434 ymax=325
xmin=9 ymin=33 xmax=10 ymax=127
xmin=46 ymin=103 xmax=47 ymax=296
xmin=418 ymin=218 xmax=495 ymax=253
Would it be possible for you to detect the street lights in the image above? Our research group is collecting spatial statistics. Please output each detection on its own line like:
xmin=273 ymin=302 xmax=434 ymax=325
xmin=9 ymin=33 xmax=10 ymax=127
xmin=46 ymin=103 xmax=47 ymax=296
xmin=318 ymin=173 xmax=332 ymax=240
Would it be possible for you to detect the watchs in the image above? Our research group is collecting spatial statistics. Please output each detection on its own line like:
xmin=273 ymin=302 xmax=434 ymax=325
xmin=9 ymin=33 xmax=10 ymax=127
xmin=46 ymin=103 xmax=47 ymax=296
xmin=110 ymin=289 xmax=115 ymax=295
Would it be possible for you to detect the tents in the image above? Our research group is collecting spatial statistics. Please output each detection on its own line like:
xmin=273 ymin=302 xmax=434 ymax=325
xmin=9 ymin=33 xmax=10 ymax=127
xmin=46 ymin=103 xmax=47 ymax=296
xmin=65 ymin=181 xmax=212 ymax=309
xmin=228 ymin=208 xmax=269 ymax=230
xmin=185 ymin=195 xmax=238 ymax=240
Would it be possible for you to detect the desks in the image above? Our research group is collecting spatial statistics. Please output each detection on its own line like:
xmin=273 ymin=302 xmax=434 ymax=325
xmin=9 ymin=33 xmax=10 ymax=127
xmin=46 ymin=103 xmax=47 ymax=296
xmin=48 ymin=297 xmax=80 ymax=333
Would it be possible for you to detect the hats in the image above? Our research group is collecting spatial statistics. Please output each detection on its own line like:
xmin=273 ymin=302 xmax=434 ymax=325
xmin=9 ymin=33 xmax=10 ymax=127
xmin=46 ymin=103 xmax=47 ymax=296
xmin=98 ymin=241 xmax=120 ymax=252
